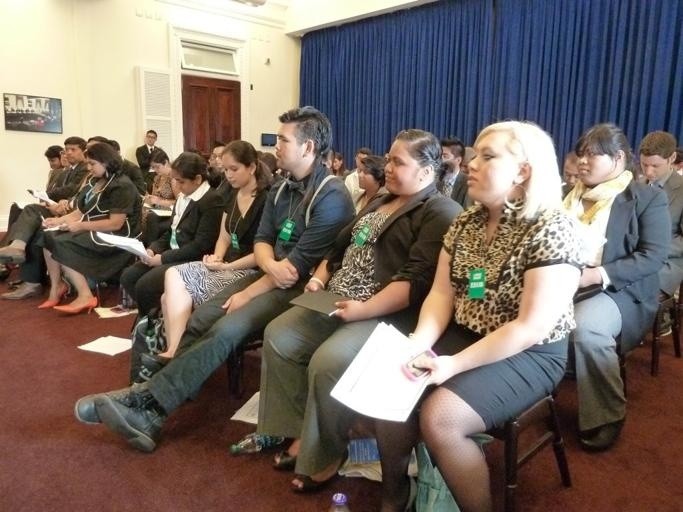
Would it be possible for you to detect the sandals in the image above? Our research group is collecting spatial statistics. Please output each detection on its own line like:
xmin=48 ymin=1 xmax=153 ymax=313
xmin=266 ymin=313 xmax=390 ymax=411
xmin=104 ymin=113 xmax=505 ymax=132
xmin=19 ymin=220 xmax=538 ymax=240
xmin=272 ymin=439 xmax=303 ymax=471
xmin=288 ymin=447 xmax=349 ymax=493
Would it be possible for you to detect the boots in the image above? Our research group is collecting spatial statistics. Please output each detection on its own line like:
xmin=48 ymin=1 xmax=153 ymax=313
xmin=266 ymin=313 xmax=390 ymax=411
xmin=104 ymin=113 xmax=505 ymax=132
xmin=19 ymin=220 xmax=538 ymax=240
xmin=96 ymin=394 xmax=168 ymax=452
xmin=73 ymin=383 xmax=156 ymax=426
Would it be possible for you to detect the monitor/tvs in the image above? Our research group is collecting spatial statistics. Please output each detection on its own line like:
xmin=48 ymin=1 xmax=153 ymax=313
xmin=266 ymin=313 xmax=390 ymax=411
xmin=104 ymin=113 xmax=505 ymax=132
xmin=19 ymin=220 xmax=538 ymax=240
xmin=262 ymin=133 xmax=277 ymax=146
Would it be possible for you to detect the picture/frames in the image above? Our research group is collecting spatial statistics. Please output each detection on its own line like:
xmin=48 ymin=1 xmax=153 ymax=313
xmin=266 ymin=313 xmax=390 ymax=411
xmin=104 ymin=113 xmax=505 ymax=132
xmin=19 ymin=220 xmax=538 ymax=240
xmin=4 ymin=93 xmax=63 ymax=133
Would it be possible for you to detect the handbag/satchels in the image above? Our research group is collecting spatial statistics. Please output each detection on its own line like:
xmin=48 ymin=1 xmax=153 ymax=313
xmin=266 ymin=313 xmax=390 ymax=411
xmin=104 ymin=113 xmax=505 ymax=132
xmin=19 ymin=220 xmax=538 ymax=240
xmin=414 ymin=432 xmax=497 ymax=512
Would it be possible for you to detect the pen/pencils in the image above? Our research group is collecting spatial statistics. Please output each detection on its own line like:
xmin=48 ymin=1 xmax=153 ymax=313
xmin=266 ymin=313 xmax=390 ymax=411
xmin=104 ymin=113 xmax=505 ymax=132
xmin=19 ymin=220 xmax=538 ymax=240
xmin=38 ymin=214 xmax=48 ymax=223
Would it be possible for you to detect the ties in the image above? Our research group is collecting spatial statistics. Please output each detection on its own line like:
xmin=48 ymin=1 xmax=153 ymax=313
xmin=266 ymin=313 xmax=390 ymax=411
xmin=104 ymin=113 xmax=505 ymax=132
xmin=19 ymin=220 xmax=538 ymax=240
xmin=651 ymin=183 xmax=659 ymax=188
xmin=150 ymin=147 xmax=153 ymax=153
xmin=64 ymin=167 xmax=74 ymax=186
xmin=442 ymin=181 xmax=451 ymax=198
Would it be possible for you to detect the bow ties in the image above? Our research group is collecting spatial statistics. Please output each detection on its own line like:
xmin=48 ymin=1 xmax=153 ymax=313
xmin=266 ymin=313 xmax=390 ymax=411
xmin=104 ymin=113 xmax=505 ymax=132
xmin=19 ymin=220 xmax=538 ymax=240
xmin=283 ymin=177 xmax=307 ymax=196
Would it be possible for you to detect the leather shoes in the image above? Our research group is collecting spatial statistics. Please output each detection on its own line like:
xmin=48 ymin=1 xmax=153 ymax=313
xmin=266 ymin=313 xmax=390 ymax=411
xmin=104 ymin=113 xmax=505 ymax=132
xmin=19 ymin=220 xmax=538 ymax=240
xmin=0 ymin=246 xmax=26 ymax=265
xmin=139 ymin=348 xmax=174 ymax=372
xmin=0 ymin=281 xmax=43 ymax=301
xmin=580 ymin=408 xmax=625 ymax=451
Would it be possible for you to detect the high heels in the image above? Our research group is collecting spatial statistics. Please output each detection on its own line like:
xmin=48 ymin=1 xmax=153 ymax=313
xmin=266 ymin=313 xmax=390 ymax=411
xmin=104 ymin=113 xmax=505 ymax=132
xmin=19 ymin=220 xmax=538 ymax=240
xmin=53 ymin=295 xmax=98 ymax=315
xmin=38 ymin=280 xmax=69 ymax=309
xmin=403 ymin=474 xmax=417 ymax=512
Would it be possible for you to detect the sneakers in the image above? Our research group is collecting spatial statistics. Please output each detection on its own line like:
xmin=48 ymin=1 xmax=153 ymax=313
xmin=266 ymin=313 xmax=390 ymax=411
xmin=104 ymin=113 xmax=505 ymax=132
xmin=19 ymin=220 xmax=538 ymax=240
xmin=658 ymin=309 xmax=673 ymax=338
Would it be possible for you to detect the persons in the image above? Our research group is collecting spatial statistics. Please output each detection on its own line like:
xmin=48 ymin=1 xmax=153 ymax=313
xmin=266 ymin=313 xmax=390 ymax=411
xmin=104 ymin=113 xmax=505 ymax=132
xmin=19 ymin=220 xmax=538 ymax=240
xmin=0 ymin=107 xmax=683 ymax=511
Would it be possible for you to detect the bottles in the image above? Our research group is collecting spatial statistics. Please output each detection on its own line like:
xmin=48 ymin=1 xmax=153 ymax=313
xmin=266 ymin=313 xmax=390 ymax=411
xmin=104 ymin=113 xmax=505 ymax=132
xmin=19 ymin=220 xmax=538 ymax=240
xmin=327 ymin=492 xmax=351 ymax=512
xmin=228 ymin=430 xmax=284 ymax=453
